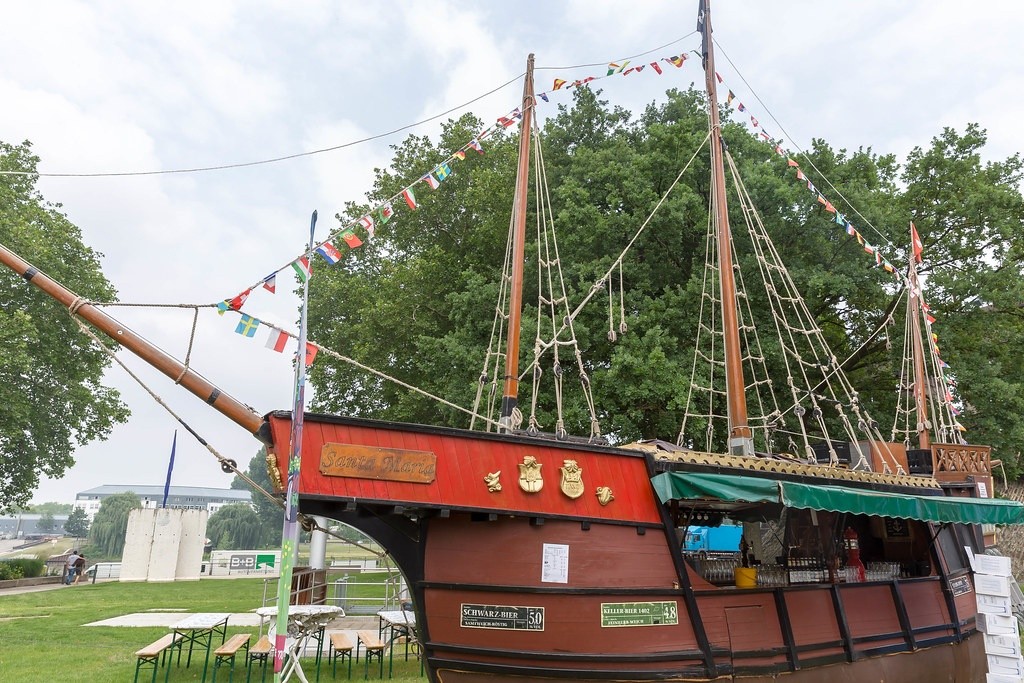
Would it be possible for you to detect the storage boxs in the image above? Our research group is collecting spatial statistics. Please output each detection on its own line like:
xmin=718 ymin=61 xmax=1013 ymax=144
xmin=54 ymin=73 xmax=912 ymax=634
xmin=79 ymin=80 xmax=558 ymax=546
xmin=986 ymin=654 xmax=1024 ymax=676
xmin=973 ymin=573 xmax=1011 ymax=597
xmin=975 ymin=594 xmax=1013 ymax=617
xmin=982 ymin=633 xmax=1021 ymax=658
xmin=974 ymin=554 xmax=1012 ymax=577
xmin=975 ymin=613 xmax=1019 ymax=636
xmin=986 ymin=673 xmax=1024 ymax=683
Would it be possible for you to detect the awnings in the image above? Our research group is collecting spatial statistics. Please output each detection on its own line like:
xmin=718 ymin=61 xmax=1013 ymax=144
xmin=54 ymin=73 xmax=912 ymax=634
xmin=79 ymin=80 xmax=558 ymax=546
xmin=651 ymin=470 xmax=1023 ymax=528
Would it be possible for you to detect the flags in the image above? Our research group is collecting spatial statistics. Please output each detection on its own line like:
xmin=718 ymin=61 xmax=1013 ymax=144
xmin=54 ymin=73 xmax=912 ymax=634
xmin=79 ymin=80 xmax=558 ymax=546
xmin=216 ymin=53 xmax=969 ymax=446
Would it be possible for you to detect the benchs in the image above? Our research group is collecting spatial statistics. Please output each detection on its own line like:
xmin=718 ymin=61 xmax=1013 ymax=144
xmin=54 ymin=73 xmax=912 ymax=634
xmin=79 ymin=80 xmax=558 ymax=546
xmin=247 ymin=634 xmax=275 ymax=683
xmin=211 ymin=634 xmax=251 ymax=683
xmin=328 ymin=632 xmax=354 ymax=681
xmin=356 ymin=630 xmax=386 ymax=680
xmin=133 ymin=633 xmax=187 ymax=683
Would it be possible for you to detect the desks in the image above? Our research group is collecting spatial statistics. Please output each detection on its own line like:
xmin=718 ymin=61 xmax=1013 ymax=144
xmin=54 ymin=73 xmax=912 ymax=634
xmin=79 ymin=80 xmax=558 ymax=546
xmin=377 ymin=611 xmax=424 ymax=680
xmin=258 ymin=605 xmax=343 ymax=683
xmin=165 ymin=611 xmax=232 ymax=683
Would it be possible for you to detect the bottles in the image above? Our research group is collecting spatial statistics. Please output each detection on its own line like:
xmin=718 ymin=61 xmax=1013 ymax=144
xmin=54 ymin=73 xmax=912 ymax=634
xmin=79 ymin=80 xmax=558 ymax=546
xmin=787 ymin=557 xmax=861 ymax=584
xmin=747 ymin=541 xmax=755 ymax=567
xmin=902 ymin=564 xmax=911 ymax=578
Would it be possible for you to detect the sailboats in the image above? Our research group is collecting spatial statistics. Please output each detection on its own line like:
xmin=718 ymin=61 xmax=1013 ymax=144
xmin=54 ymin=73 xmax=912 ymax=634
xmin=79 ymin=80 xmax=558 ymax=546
xmin=0 ymin=0 xmax=1024 ymax=683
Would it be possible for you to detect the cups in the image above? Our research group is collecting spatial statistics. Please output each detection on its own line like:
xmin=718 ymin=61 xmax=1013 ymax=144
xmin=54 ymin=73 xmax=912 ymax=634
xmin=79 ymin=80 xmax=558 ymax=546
xmin=864 ymin=561 xmax=901 ymax=581
xmin=703 ymin=551 xmax=785 ymax=585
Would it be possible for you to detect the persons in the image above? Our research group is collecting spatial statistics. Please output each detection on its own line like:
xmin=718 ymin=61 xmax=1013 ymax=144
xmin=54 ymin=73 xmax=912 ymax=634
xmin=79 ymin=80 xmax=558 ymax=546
xmin=66 ymin=551 xmax=86 ymax=585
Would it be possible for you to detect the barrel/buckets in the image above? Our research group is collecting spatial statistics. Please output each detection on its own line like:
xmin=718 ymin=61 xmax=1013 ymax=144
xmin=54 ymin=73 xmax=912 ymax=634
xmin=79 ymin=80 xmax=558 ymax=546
xmin=734 ymin=567 xmax=757 ymax=587
